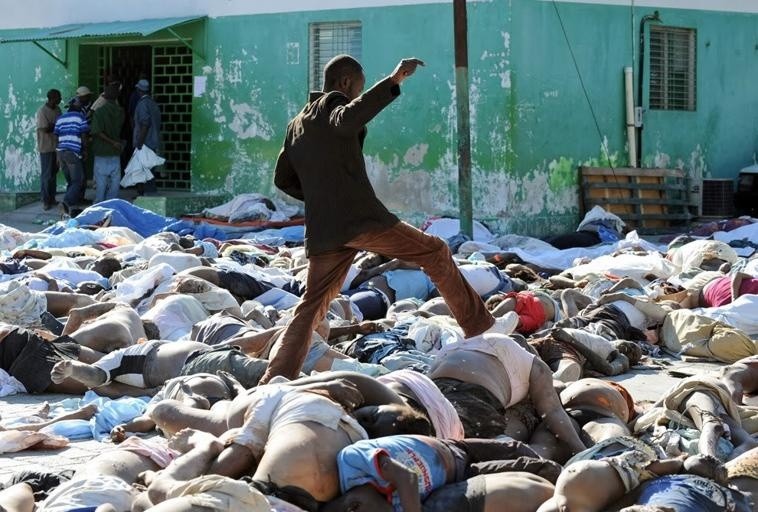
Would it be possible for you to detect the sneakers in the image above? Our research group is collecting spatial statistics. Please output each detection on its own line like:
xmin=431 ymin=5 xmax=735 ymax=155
xmin=482 ymin=309 xmax=520 ymax=336
xmin=44 ymin=200 xmax=69 ymax=214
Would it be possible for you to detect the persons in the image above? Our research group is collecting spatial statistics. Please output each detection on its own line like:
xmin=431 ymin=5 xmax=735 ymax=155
xmin=90 ymin=85 xmax=130 ymax=204
xmin=67 ymin=85 xmax=96 ymax=141
xmin=131 ymin=79 xmax=162 ymax=199
xmin=36 ymin=88 xmax=63 ymax=210
xmin=258 ymin=53 xmax=521 ymax=385
xmin=127 ymin=70 xmax=150 ymax=128
xmin=90 ymin=73 xmax=122 ymax=112
xmin=53 ymin=96 xmax=90 ymax=212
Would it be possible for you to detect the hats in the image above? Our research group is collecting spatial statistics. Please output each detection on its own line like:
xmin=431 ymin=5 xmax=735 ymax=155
xmin=75 ymin=86 xmax=94 ymax=97
xmin=135 ymin=79 xmax=150 ymax=92
